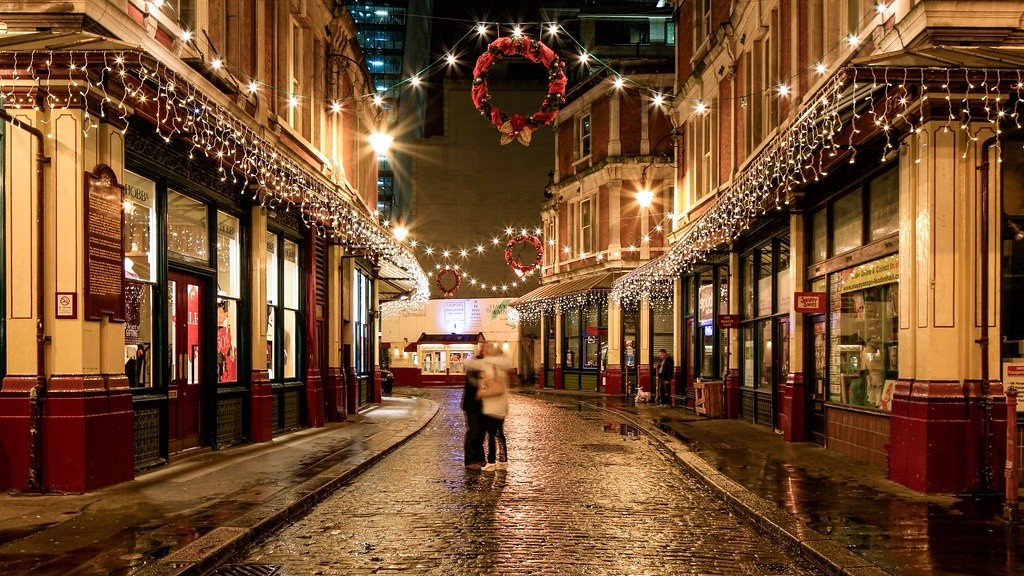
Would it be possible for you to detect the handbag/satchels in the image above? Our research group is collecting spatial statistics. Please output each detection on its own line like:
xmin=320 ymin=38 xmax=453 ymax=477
xmin=477 ymin=362 xmax=504 ymax=399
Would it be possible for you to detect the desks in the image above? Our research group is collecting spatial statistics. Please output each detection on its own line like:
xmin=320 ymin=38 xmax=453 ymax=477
xmin=693 ymin=381 xmax=723 ymax=418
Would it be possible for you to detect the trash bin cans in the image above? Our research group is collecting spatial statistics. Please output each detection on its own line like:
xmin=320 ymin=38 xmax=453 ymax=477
xmin=381 ymin=370 xmax=395 ymax=394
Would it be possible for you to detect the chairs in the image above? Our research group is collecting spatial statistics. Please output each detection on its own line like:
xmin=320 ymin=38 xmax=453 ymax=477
xmin=670 ymin=379 xmax=713 ymax=415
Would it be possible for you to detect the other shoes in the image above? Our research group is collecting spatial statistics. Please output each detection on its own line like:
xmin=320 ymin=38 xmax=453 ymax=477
xmin=496 ymin=462 xmax=507 ymax=470
xmin=662 ymin=404 xmax=671 ymax=408
xmin=657 ymin=404 xmax=663 ymax=408
xmin=468 ymin=462 xmax=486 ymax=470
xmin=481 ymin=463 xmax=496 ymax=472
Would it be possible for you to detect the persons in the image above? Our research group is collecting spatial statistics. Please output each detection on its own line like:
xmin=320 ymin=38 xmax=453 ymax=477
xmin=461 ymin=345 xmax=510 ymax=471
xmin=657 ymin=349 xmax=673 ymax=408
